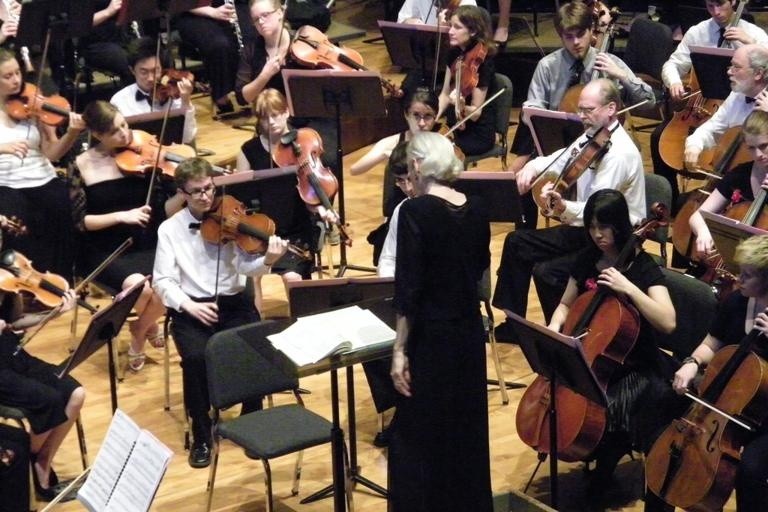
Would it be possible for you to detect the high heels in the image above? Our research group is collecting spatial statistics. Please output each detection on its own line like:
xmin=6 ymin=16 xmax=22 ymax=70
xmin=32 ymin=455 xmax=57 ymax=484
xmin=31 ymin=460 xmax=53 ymax=500
xmin=212 ymin=93 xmax=234 ymax=113
xmin=492 ymin=25 xmax=508 ymax=48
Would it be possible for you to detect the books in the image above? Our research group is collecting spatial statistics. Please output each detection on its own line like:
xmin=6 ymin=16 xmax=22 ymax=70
xmin=76 ymin=408 xmax=175 ymax=512
xmin=267 ymin=306 xmax=400 ymax=364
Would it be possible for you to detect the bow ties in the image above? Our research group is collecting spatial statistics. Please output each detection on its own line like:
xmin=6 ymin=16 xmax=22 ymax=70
xmin=189 ymin=223 xmax=200 ymax=229
xmin=580 ymin=122 xmax=620 ymax=148
xmin=745 ymin=96 xmax=758 ymax=104
xmin=135 ymin=90 xmax=149 ymax=101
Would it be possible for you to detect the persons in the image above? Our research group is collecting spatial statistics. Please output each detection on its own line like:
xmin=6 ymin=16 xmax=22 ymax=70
xmin=649 ymin=0 xmax=767 ymax=218
xmin=234 ymin=0 xmax=340 ymax=137
xmin=394 ymin=0 xmax=481 ymax=96
xmin=69 ymin=101 xmax=189 ymax=371
xmin=151 ymin=159 xmax=291 ymax=468
xmin=110 ymin=37 xmax=198 ymax=147
xmin=64 ymin=0 xmax=156 ymax=90
xmin=434 ymin=6 xmax=501 ymax=157
xmin=0 ymin=47 xmax=87 ymax=292
xmin=162 ymin=0 xmax=242 ymax=119
xmin=356 ymin=140 xmax=458 ymax=449
xmin=484 ymin=79 xmax=648 ymax=344
xmin=350 ymin=90 xmax=463 ymax=268
xmin=642 ymin=236 xmax=767 ymax=512
xmin=283 ymin=0 xmax=333 ymax=33
xmin=514 ymin=1 xmax=656 ymax=226
xmin=1 ymin=0 xmax=22 ymax=46
xmin=542 ymin=189 xmax=677 ymax=463
xmin=0 ymin=307 xmax=86 ymax=503
xmin=685 ymin=44 xmax=768 ymax=169
xmin=236 ymin=90 xmax=340 ymax=282
xmin=387 ymin=133 xmax=492 ymax=511
xmin=685 ymin=111 xmax=767 ymax=258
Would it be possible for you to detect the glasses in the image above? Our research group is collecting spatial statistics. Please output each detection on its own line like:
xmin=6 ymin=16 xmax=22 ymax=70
xmin=408 ymin=111 xmax=433 ymax=121
xmin=396 ymin=177 xmax=413 ymax=187
xmin=182 ymin=182 xmax=216 ymax=200
xmin=577 ymin=102 xmax=609 ymax=115
xmin=251 ymin=8 xmax=277 ymax=25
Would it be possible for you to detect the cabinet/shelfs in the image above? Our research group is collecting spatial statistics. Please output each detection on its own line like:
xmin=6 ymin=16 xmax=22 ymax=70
xmin=294 ymin=304 xmax=398 ymax=512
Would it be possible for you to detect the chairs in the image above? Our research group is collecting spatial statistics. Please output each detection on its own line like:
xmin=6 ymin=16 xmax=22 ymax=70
xmin=644 ymin=173 xmax=671 ymax=267
xmin=41 ymin=20 xmax=119 ymax=111
xmin=374 ymin=267 xmax=509 ymax=436
xmin=163 ymin=277 xmax=274 ymax=450
xmin=254 ymin=115 xmax=337 ymax=279
xmin=462 ymin=73 xmax=513 ymax=171
xmin=623 ymin=18 xmax=672 ymax=122
xmin=584 ymin=267 xmax=716 ymax=495
xmin=69 ymin=205 xmax=138 ymax=382
xmin=205 ymin=316 xmax=355 ymax=511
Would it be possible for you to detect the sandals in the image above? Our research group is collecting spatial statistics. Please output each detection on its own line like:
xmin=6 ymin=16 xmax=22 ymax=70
xmin=128 ymin=343 xmax=145 ymax=370
xmin=146 ymin=324 xmax=168 ymax=349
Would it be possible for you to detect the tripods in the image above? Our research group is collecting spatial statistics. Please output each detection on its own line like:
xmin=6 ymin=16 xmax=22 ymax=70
xmin=548 ymin=355 xmax=557 ymax=509
xmin=299 ymin=369 xmax=393 ymax=511
xmin=329 ymin=367 xmax=346 ymax=512
xmin=103 ymin=325 xmax=121 ymax=416
xmin=55 ymin=13 xmax=68 ymax=138
xmin=307 ymin=86 xmax=378 ymax=278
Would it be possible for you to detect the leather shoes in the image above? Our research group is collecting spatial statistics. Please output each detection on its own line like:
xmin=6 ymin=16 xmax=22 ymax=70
xmin=484 ymin=323 xmax=518 ymax=344
xmin=190 ymin=440 xmax=212 ymax=467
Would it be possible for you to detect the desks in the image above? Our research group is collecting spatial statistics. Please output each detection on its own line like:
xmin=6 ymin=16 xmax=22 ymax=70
xmin=172 ymin=30 xmax=217 ymax=120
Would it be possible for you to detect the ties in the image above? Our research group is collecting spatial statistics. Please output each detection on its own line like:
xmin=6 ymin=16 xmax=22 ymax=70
xmin=564 ymin=62 xmax=582 ymax=97
xmin=718 ymin=28 xmax=726 ymax=51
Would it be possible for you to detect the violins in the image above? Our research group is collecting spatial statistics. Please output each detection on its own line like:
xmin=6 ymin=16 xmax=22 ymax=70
xmin=432 ymin=120 xmax=467 ymax=164
xmin=450 ymin=41 xmax=490 ymax=132
xmin=113 ymin=128 xmax=233 ymax=182
xmin=270 ymin=126 xmax=353 ymax=247
xmin=287 ymin=24 xmax=406 ymax=100
xmin=3 ymin=81 xmax=73 ymax=127
xmin=149 ymin=68 xmax=213 ymax=107
xmin=200 ymin=194 xmax=313 ymax=265
xmin=540 ymin=125 xmax=613 ymax=217
xmin=433 ymin=0 xmax=463 ymax=24
xmin=1 ymin=247 xmax=101 ymax=316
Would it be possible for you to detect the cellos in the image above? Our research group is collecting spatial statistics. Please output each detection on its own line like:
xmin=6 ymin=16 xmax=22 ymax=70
xmin=643 ymin=313 xmax=764 ymax=512
xmin=515 ymin=200 xmax=670 ymax=464
xmin=658 ymin=0 xmax=755 ymax=178
xmin=700 ymin=187 xmax=764 ymax=304
xmin=672 ymin=90 xmax=764 ymax=268
xmin=555 ymin=6 xmax=627 ymax=153
